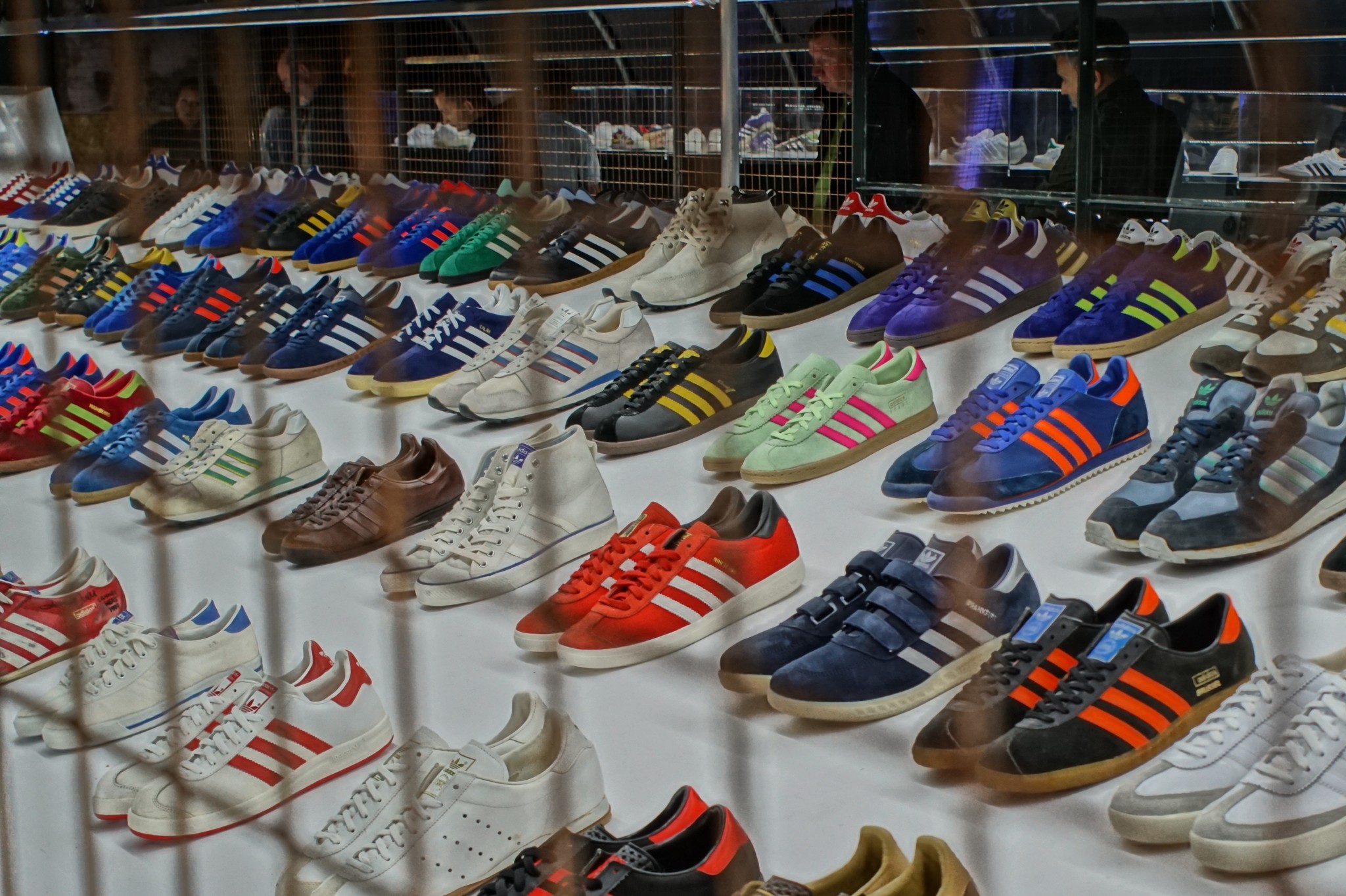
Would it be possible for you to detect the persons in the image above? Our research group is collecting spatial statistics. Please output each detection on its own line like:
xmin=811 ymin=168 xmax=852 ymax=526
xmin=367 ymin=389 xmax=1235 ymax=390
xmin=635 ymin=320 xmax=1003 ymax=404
xmin=1044 ymin=16 xmax=1164 ymax=228
xmin=145 ymin=81 xmax=222 ymax=170
xmin=254 ymin=41 xmax=353 ymax=175
xmin=804 ymin=4 xmax=936 ymax=214
xmin=531 ymin=71 xmax=605 ymax=195
xmin=432 ymin=73 xmax=507 ymax=185
xmin=336 ymin=50 xmax=403 ymax=175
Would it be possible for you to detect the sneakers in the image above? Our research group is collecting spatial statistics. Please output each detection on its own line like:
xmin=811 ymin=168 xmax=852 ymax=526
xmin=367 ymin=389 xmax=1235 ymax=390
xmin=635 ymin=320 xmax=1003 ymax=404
xmin=718 ymin=528 xmax=984 ymax=695
xmin=767 ymin=534 xmax=1043 ymax=723
xmin=552 ymin=804 xmax=767 ymax=896
xmin=514 ymin=487 xmax=750 ymax=653
xmin=471 ymin=784 xmax=711 ymax=896
xmin=911 ymin=576 xmax=1172 ymax=769
xmin=93 ymin=638 xmax=336 ymax=821
xmin=729 ymin=825 xmax=912 ymax=896
xmin=974 ymin=592 xmax=1259 ymax=797
xmin=0 ymin=545 xmax=91 ymax=596
xmin=1107 ymin=645 xmax=1345 ymax=845
xmin=128 ymin=648 xmax=394 ymax=846
xmin=41 ymin=602 xmax=265 ymax=751
xmin=272 ymin=691 xmax=548 ymax=896
xmin=13 ymin=596 xmax=222 ymax=739
xmin=554 ymin=488 xmax=807 ymax=668
xmin=862 ymin=835 xmax=981 ymax=895
xmin=0 ymin=103 xmax=1344 ymax=618
xmin=1189 ymin=667 xmax=1346 ymax=871
xmin=309 ymin=706 xmax=612 ymax=895
xmin=0 ymin=555 xmax=127 ymax=687
